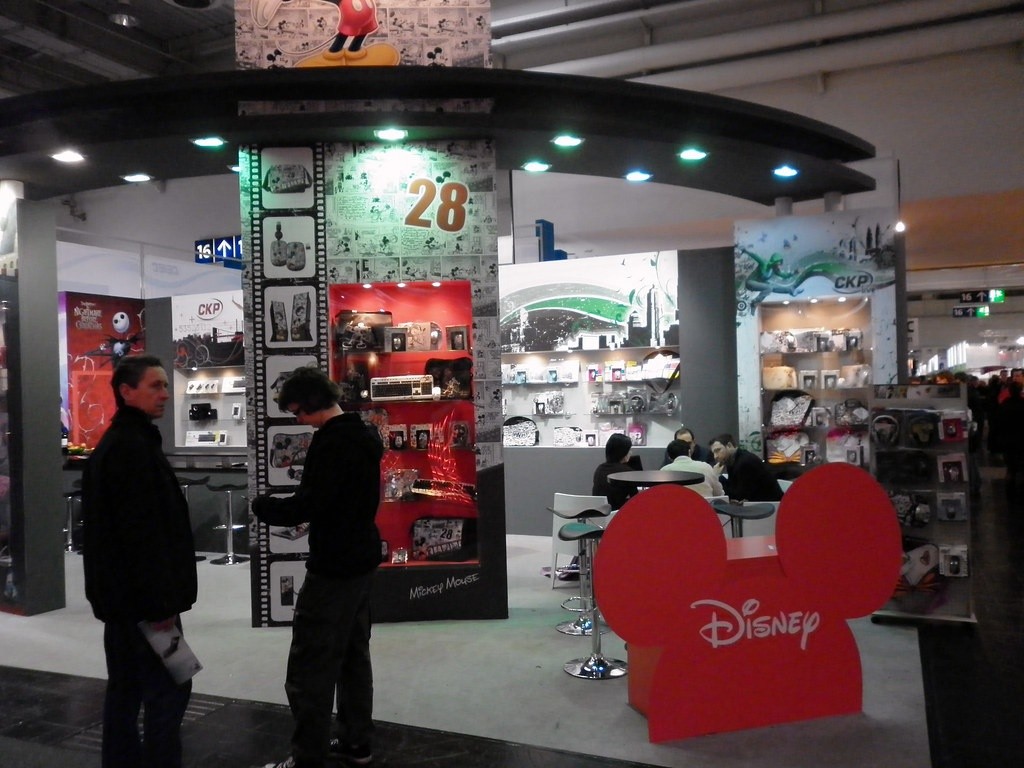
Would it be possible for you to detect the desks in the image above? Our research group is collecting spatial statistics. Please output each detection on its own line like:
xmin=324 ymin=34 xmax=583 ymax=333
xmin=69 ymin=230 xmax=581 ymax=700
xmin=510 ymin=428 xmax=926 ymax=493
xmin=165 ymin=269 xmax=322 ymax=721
xmin=607 ymin=470 xmax=706 ymax=651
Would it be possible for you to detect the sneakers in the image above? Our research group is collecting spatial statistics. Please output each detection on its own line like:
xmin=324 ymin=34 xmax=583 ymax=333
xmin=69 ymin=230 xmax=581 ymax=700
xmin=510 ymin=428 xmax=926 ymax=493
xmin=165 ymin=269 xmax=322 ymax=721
xmin=327 ymin=737 xmax=371 ymax=764
xmin=264 ymin=756 xmax=302 ymax=768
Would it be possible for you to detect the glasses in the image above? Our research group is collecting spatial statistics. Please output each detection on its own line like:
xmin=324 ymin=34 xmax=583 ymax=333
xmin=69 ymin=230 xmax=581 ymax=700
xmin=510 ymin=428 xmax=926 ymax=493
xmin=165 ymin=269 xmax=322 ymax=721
xmin=293 ymin=407 xmax=301 ymax=417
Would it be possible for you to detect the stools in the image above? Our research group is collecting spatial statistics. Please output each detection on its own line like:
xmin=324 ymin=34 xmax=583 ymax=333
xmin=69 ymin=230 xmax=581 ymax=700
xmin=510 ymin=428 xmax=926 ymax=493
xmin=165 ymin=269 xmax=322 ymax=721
xmin=63 ymin=490 xmax=82 ymax=552
xmin=546 ymin=504 xmax=613 ymax=636
xmin=558 ymin=523 xmax=629 ymax=680
xmin=205 ymin=482 xmax=250 ymax=566
xmin=709 ymin=499 xmax=775 ymax=539
xmin=176 ymin=476 xmax=211 ymax=562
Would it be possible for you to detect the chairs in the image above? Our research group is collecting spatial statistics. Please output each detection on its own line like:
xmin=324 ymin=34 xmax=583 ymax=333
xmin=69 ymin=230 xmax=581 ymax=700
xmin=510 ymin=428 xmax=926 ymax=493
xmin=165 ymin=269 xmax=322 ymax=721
xmin=550 ymin=492 xmax=619 ymax=590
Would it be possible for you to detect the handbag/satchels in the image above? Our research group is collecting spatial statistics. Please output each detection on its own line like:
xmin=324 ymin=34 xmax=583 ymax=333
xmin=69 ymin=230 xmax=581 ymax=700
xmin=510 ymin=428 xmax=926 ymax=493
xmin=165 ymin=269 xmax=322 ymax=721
xmin=558 ymin=543 xmax=590 ymax=581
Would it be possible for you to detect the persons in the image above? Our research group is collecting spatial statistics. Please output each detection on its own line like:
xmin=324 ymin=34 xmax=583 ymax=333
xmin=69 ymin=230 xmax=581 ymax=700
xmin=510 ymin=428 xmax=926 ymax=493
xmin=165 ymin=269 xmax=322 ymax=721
xmin=81 ymin=354 xmax=199 ymax=768
xmin=593 ymin=432 xmax=643 ymax=511
xmin=657 ymin=428 xmax=725 ymax=497
xmin=908 ymin=369 xmax=1024 ymax=469
xmin=252 ymin=365 xmax=386 ymax=768
xmin=709 ymin=432 xmax=784 ymax=501
